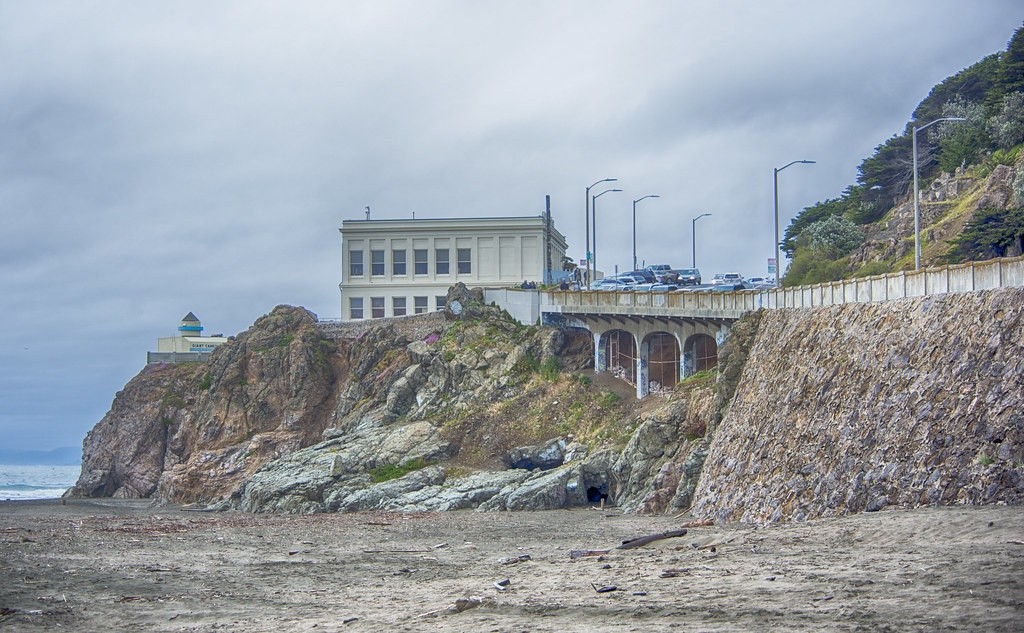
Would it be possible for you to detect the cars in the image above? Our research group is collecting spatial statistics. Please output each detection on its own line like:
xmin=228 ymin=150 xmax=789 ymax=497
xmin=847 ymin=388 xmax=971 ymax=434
xmin=580 ymin=263 xmax=777 ymax=292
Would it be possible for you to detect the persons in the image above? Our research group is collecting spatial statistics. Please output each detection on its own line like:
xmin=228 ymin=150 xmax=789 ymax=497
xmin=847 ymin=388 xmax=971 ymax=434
xmin=584 ymin=272 xmax=586 ymax=285
xmin=560 ymin=280 xmax=568 ymax=290
xmin=521 ymin=280 xmax=536 ymax=289
xmin=574 ymin=268 xmax=578 ymax=281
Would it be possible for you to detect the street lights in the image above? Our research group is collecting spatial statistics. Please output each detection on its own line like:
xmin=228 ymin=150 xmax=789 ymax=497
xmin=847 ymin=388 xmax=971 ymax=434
xmin=692 ymin=213 xmax=713 ymax=267
xmin=632 ymin=195 xmax=660 ymax=271
xmin=911 ymin=118 xmax=967 ymax=269
xmin=584 ymin=177 xmax=618 ymax=292
xmin=774 ymin=160 xmax=816 ymax=285
xmin=593 ymin=189 xmax=623 ymax=282
